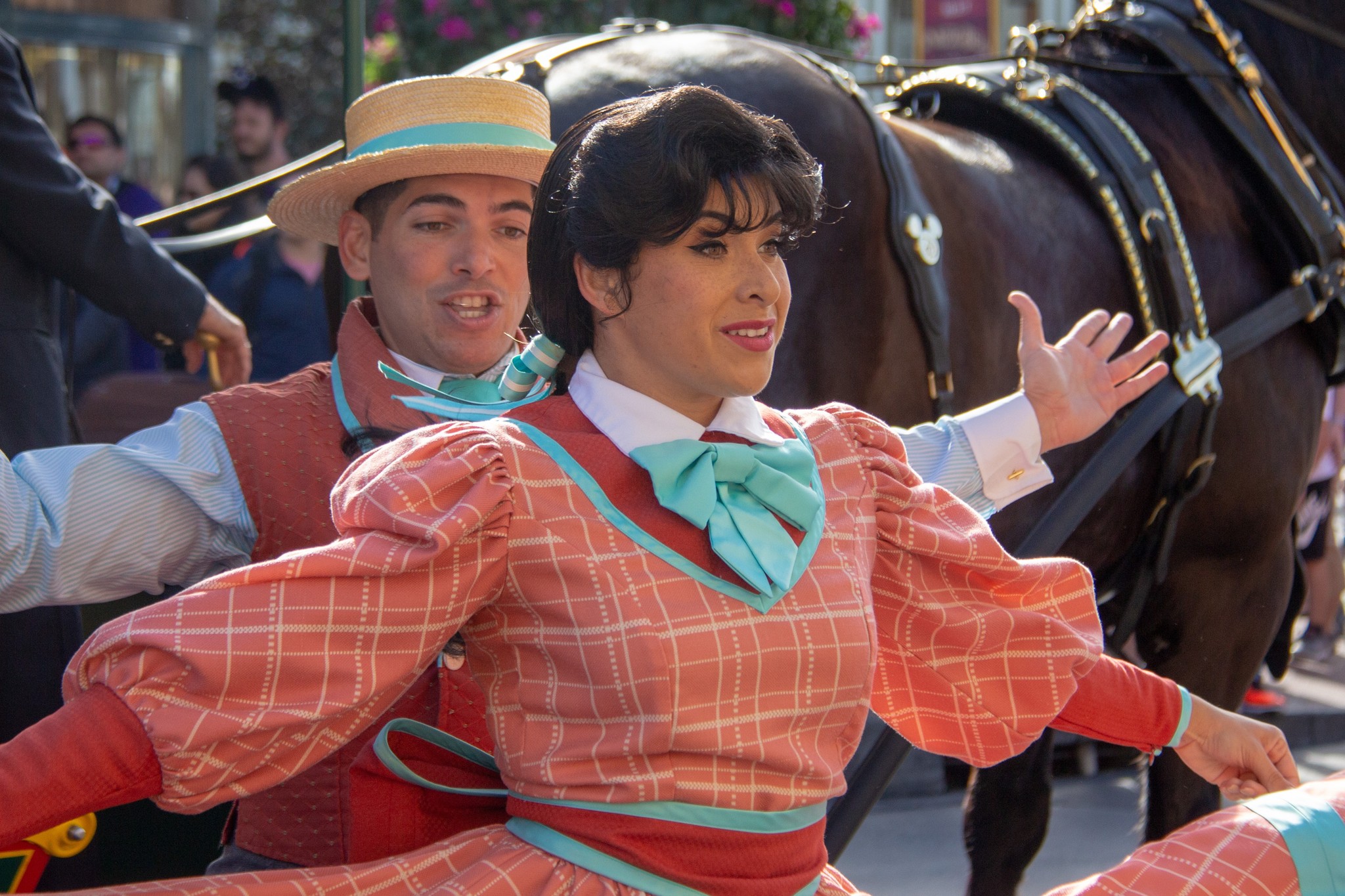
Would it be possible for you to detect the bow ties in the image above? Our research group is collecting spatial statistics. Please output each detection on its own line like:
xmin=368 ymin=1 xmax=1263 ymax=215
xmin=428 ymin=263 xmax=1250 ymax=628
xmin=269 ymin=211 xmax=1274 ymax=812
xmin=628 ymin=437 xmax=822 ymax=599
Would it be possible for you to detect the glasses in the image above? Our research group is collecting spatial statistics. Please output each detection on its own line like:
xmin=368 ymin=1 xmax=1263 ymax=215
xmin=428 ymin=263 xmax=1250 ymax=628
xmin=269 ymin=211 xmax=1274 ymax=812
xmin=65 ymin=137 xmax=113 ymax=152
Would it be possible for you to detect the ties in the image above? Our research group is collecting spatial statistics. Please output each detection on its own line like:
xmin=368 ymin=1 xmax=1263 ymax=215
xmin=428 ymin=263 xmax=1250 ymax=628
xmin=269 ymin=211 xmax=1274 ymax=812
xmin=439 ymin=372 xmax=505 ymax=404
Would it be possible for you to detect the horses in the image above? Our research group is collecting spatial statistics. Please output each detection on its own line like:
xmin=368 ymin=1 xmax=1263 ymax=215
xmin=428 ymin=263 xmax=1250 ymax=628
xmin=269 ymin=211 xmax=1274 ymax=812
xmin=444 ymin=0 xmax=1344 ymax=896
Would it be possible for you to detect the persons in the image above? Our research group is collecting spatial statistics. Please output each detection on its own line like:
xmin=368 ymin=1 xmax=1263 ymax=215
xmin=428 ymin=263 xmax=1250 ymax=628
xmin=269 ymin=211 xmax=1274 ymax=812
xmin=1 ymin=83 xmax=1345 ymax=896
xmin=0 ymin=75 xmax=1169 ymax=881
xmin=172 ymin=77 xmax=332 ymax=384
xmin=939 ymin=361 xmax=1344 ymax=787
xmin=0 ymin=30 xmax=253 ymax=460
xmin=63 ymin=114 xmax=175 ymax=374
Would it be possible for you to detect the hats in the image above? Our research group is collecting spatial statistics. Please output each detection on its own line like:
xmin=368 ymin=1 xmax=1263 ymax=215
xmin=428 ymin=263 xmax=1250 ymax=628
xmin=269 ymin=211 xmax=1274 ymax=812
xmin=267 ymin=73 xmax=559 ymax=247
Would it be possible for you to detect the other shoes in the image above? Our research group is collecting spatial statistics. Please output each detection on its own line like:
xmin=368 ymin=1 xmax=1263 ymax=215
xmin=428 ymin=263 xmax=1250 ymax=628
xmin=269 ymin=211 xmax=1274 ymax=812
xmin=1290 ymin=606 xmax=1345 ymax=670
xmin=1241 ymin=684 xmax=1283 ymax=706
xmin=1092 ymin=740 xmax=1148 ymax=771
xmin=1050 ymin=741 xmax=1080 ymax=778
